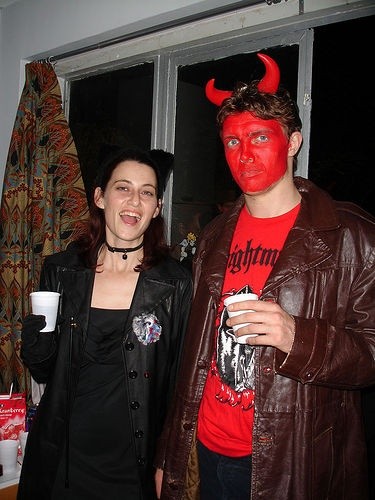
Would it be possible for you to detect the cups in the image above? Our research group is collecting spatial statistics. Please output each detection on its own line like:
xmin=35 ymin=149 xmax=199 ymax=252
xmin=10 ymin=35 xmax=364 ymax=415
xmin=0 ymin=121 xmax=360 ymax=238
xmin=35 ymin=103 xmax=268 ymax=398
xmin=20 ymin=431 xmax=29 ymax=458
xmin=223 ymin=293 xmax=259 ymax=344
xmin=0 ymin=440 xmax=20 ymax=476
xmin=29 ymin=291 xmax=61 ymax=333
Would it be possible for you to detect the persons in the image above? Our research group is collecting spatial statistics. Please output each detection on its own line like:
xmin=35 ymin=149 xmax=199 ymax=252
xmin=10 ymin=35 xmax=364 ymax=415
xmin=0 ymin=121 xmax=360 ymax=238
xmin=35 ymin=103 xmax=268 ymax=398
xmin=16 ymin=148 xmax=193 ymax=500
xmin=155 ymin=86 xmax=374 ymax=500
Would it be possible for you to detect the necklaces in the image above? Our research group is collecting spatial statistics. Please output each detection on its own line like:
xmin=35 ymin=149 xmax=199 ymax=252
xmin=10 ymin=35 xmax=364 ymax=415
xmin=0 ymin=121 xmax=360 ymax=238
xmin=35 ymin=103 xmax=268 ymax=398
xmin=103 ymin=241 xmax=144 ymax=259
